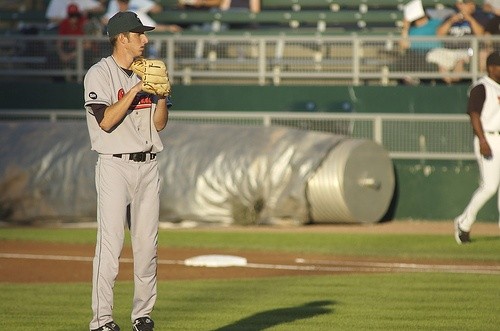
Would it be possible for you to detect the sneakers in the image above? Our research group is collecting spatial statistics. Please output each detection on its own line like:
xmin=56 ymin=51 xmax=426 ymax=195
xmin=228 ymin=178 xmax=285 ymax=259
xmin=133 ymin=317 xmax=154 ymax=331
xmin=91 ymin=321 xmax=120 ymax=331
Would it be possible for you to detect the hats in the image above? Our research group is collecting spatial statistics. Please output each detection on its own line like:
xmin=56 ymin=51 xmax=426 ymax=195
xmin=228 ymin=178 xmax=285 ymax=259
xmin=106 ymin=11 xmax=155 ymax=36
xmin=68 ymin=4 xmax=77 ymax=14
xmin=487 ymin=51 xmax=500 ymax=67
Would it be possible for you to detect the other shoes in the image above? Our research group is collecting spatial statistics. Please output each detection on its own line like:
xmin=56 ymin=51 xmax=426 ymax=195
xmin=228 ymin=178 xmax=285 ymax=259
xmin=453 ymin=217 xmax=471 ymax=246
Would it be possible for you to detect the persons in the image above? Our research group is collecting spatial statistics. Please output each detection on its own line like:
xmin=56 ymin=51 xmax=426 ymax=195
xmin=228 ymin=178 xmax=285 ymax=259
xmin=388 ymin=0 xmax=500 ymax=87
xmin=84 ymin=11 xmax=172 ymax=331
xmin=46 ymin=0 xmax=260 ymax=80
xmin=455 ymin=51 xmax=500 ymax=243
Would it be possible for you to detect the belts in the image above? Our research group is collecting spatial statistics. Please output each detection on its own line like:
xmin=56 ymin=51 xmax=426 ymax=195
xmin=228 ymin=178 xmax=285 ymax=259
xmin=488 ymin=131 xmax=500 ymax=135
xmin=113 ymin=152 xmax=156 ymax=162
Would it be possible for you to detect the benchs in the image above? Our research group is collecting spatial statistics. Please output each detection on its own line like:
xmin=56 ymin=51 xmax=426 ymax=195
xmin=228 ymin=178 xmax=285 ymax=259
xmin=0 ymin=0 xmax=484 ymax=70
